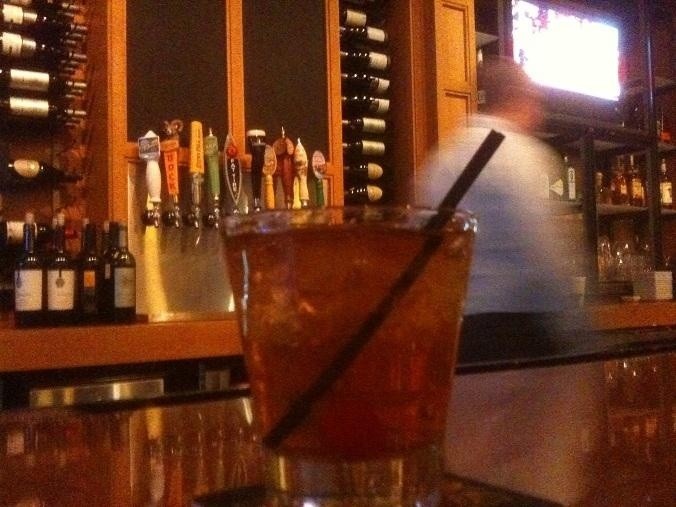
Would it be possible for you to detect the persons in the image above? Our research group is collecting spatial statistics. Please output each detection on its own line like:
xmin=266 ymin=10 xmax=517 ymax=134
xmin=422 ymin=55 xmax=578 ymax=366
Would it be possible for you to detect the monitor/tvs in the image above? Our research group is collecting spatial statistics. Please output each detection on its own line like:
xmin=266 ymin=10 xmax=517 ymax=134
xmin=497 ymin=0 xmax=644 ymax=129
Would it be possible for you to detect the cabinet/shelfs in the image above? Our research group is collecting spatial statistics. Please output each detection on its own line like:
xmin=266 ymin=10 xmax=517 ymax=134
xmin=0 ymin=0 xmax=675 ymax=392
